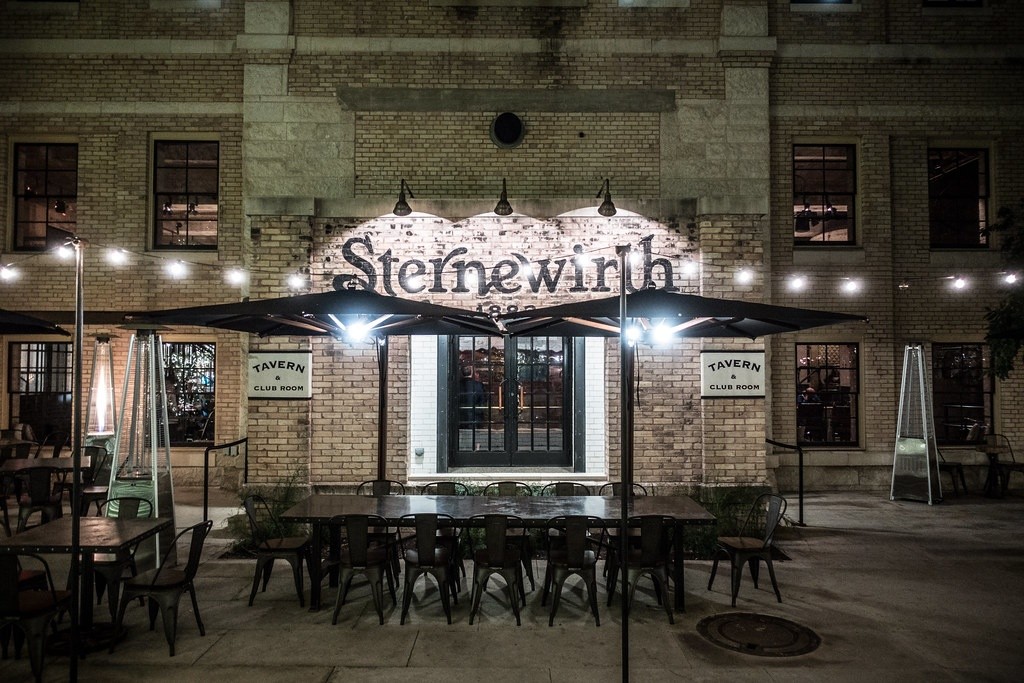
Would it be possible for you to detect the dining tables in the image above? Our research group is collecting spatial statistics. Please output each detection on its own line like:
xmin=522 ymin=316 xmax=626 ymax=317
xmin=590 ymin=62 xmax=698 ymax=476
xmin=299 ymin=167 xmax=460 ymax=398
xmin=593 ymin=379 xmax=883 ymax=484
xmin=1 ymin=438 xmax=40 ymax=500
xmin=278 ymin=494 xmax=716 ymax=611
xmin=0 ymin=455 xmax=91 ymax=523
xmin=0 ymin=514 xmax=172 ymax=658
xmin=53 ymin=444 xmax=107 ymax=516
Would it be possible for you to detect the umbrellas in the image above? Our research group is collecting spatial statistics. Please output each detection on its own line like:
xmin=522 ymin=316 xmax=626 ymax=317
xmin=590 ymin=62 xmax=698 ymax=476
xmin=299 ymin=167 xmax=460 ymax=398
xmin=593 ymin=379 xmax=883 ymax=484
xmin=126 ymin=283 xmax=864 ymax=497
xmin=0 ymin=309 xmax=71 ymax=339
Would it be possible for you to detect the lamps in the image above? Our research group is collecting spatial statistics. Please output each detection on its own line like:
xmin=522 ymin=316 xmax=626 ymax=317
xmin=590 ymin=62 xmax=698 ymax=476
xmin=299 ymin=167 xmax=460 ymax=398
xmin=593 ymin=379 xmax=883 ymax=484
xmin=595 ymin=178 xmax=617 ymax=217
xmin=494 ymin=177 xmax=513 ymax=216
xmin=393 ymin=178 xmax=415 ymax=215
xmin=80 ymin=333 xmax=116 ymax=456
xmin=105 ymin=329 xmax=178 ymax=568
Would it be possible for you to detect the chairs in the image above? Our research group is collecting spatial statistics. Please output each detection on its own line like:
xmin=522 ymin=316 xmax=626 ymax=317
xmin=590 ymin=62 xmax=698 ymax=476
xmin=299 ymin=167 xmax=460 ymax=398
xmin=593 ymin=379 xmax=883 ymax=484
xmin=598 ymin=481 xmax=648 ymax=590
xmin=937 ymin=447 xmax=969 ymax=497
xmin=81 ymin=453 xmax=129 ymax=516
xmin=541 ymin=481 xmax=592 ymax=556
xmin=0 ymin=520 xmax=59 ymax=634
xmin=244 ymin=494 xmax=312 ymax=607
xmin=542 ymin=514 xmax=605 ymax=626
xmin=467 ymin=513 xmax=526 ymax=625
xmin=606 ymin=514 xmax=677 ymax=624
xmin=1 ymin=550 xmax=81 ymax=682
xmin=979 ymin=433 xmax=1024 ymax=499
xmin=356 ymin=479 xmax=406 ymax=587
xmin=398 ymin=513 xmax=458 ymax=624
xmin=66 ymin=496 xmax=153 ymax=606
xmin=483 ymin=481 xmax=535 ymax=591
xmin=14 ymin=465 xmax=66 ymax=533
xmin=35 ymin=430 xmax=69 ymax=480
xmin=708 ymin=492 xmax=787 ymax=607
xmin=421 ymin=481 xmax=468 ymax=577
xmin=108 ymin=519 xmax=213 ymax=656
xmin=329 ymin=512 xmax=397 ymax=624
xmin=0 ymin=428 xmax=26 ymax=466
